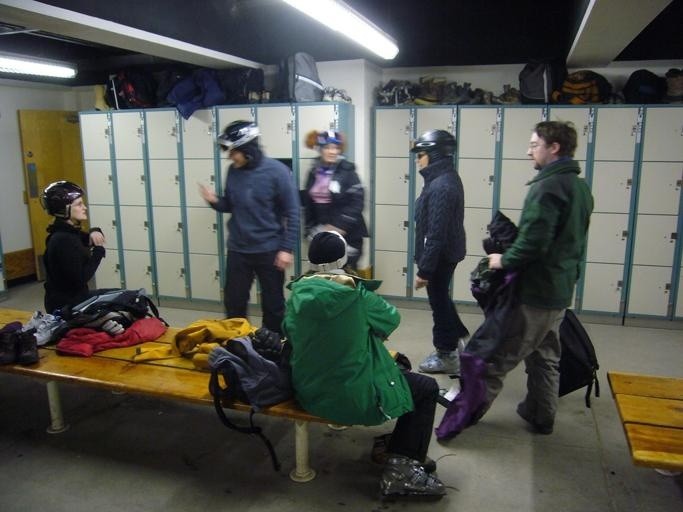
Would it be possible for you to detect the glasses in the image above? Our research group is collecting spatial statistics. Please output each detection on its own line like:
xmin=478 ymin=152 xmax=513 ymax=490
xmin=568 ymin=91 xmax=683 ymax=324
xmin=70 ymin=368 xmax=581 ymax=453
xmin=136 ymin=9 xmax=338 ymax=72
xmin=417 ymin=153 xmax=427 ymax=159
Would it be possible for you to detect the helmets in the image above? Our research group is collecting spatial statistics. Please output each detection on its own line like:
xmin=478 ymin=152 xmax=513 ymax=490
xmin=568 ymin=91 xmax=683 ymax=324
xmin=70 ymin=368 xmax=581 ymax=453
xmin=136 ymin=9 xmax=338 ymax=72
xmin=409 ymin=129 xmax=457 ymax=159
xmin=43 ymin=179 xmax=84 ymax=219
xmin=217 ymin=119 xmax=259 ymax=149
xmin=315 ymin=129 xmax=344 ymax=146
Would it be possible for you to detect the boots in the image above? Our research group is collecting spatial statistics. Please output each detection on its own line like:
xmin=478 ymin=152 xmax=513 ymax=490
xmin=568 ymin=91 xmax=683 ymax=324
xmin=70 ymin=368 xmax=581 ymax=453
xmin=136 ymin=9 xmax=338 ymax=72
xmin=93 ymin=84 xmax=110 ymax=112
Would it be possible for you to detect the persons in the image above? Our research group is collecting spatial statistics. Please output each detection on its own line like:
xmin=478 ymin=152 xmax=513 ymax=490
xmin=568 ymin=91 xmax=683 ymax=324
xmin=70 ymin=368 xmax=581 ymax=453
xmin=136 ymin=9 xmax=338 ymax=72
xmin=196 ymin=119 xmax=301 ymax=336
xmin=39 ymin=180 xmax=110 ymax=315
xmin=301 ymin=130 xmax=369 ymax=276
xmin=285 ymin=226 xmax=449 ymax=500
xmin=457 ymin=120 xmax=594 ymax=435
xmin=405 ymin=127 xmax=477 ymax=375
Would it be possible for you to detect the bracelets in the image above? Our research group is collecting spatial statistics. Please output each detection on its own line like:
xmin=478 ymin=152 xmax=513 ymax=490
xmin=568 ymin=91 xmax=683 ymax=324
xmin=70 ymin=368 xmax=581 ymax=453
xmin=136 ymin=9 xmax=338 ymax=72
xmin=88 ymin=226 xmax=104 ymax=234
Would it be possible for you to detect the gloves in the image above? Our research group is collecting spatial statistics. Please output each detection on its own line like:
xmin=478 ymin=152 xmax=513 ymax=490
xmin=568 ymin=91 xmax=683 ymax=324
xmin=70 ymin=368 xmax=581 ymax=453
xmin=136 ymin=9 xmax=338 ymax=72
xmin=101 ymin=319 xmax=125 ymax=336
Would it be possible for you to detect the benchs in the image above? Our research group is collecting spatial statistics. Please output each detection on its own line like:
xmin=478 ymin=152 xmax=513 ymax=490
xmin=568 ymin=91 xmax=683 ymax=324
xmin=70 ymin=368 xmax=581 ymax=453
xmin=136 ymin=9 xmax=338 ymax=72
xmin=1 ymin=300 xmax=400 ymax=484
xmin=605 ymin=367 xmax=682 ymax=476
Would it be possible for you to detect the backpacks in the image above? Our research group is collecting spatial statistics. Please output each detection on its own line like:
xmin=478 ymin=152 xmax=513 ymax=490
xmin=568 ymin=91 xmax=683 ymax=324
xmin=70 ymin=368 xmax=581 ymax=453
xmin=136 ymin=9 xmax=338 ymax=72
xmin=518 ymin=54 xmax=567 ymax=103
xmin=171 ymin=316 xmax=293 ymax=414
xmin=61 ymin=286 xmax=151 ymax=329
xmin=103 ymin=51 xmax=325 ymax=120
xmin=552 ymin=67 xmax=683 ymax=106
xmin=557 ymin=308 xmax=600 ymax=398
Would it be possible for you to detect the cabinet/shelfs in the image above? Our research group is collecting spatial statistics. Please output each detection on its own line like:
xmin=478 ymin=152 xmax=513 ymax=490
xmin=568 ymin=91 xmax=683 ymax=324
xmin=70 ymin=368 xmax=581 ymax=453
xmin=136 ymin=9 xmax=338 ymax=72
xmin=78 ymin=101 xmax=357 ymax=318
xmin=366 ymin=101 xmax=682 ymax=332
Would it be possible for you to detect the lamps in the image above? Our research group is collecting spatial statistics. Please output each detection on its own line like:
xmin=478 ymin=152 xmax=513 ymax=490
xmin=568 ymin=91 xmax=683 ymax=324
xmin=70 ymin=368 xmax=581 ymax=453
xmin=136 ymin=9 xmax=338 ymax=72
xmin=1 ymin=54 xmax=78 ymax=81
xmin=271 ymin=0 xmax=398 ymax=66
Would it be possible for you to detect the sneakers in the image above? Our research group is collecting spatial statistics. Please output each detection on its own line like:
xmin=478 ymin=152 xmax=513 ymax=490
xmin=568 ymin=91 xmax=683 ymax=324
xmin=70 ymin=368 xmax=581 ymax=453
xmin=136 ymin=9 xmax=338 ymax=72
xmin=322 ymin=88 xmax=352 ymax=104
xmin=376 ymin=75 xmax=521 ymax=107
xmin=370 ymin=434 xmax=446 ymax=498
xmin=0 ymin=309 xmax=62 ymax=366
xmin=418 ymin=333 xmax=472 ymax=378
xmin=517 ymin=399 xmax=555 ymax=435
